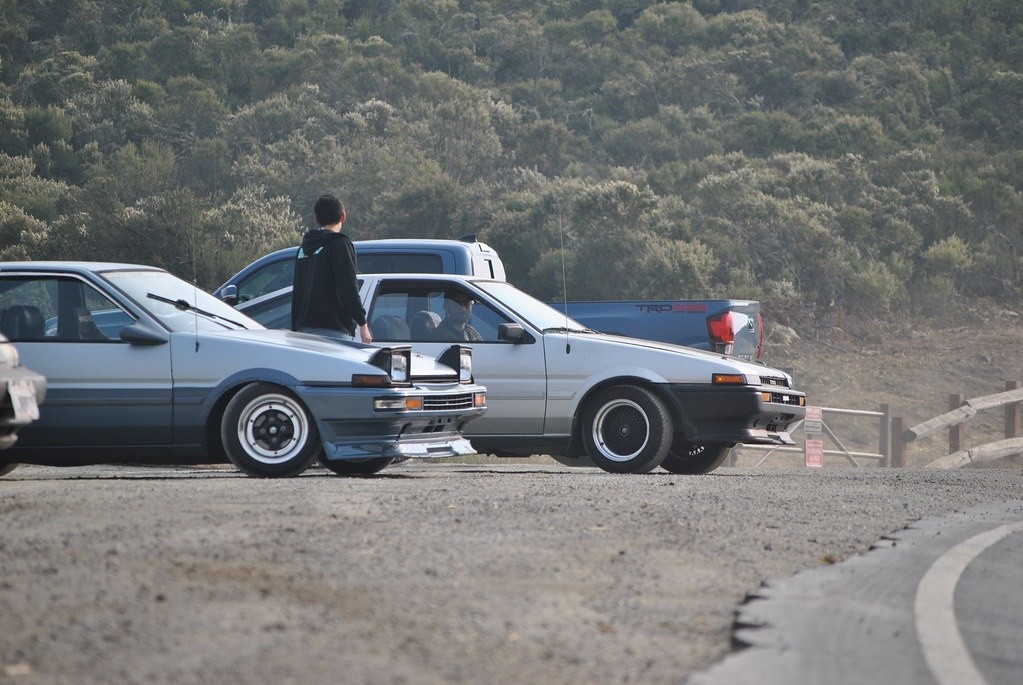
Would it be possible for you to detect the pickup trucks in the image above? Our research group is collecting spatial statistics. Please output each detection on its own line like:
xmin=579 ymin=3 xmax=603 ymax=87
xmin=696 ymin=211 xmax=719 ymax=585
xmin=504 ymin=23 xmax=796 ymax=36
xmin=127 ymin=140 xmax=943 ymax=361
xmin=43 ymin=235 xmax=762 ymax=366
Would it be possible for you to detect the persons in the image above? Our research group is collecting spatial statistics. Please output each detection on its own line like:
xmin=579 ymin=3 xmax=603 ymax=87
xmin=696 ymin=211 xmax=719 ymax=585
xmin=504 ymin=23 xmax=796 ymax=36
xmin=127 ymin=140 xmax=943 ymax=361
xmin=434 ymin=288 xmax=482 ymax=340
xmin=291 ymin=195 xmax=372 ymax=343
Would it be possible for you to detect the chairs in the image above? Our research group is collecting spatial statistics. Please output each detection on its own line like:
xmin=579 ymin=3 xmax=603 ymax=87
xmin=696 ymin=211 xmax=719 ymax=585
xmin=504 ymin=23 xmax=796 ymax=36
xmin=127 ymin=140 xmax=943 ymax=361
xmin=3 ymin=305 xmax=46 ymax=339
xmin=412 ymin=311 xmax=442 ymax=338
xmin=59 ymin=299 xmax=111 ymax=341
xmin=373 ymin=315 xmax=411 ymax=340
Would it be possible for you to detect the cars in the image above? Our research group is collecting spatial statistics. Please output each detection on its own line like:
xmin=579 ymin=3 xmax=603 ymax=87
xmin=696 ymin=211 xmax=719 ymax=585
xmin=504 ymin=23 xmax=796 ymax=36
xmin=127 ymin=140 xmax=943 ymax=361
xmin=229 ymin=274 xmax=806 ymax=480
xmin=0 ymin=257 xmax=491 ymax=479
xmin=0 ymin=331 xmax=46 ymax=452
